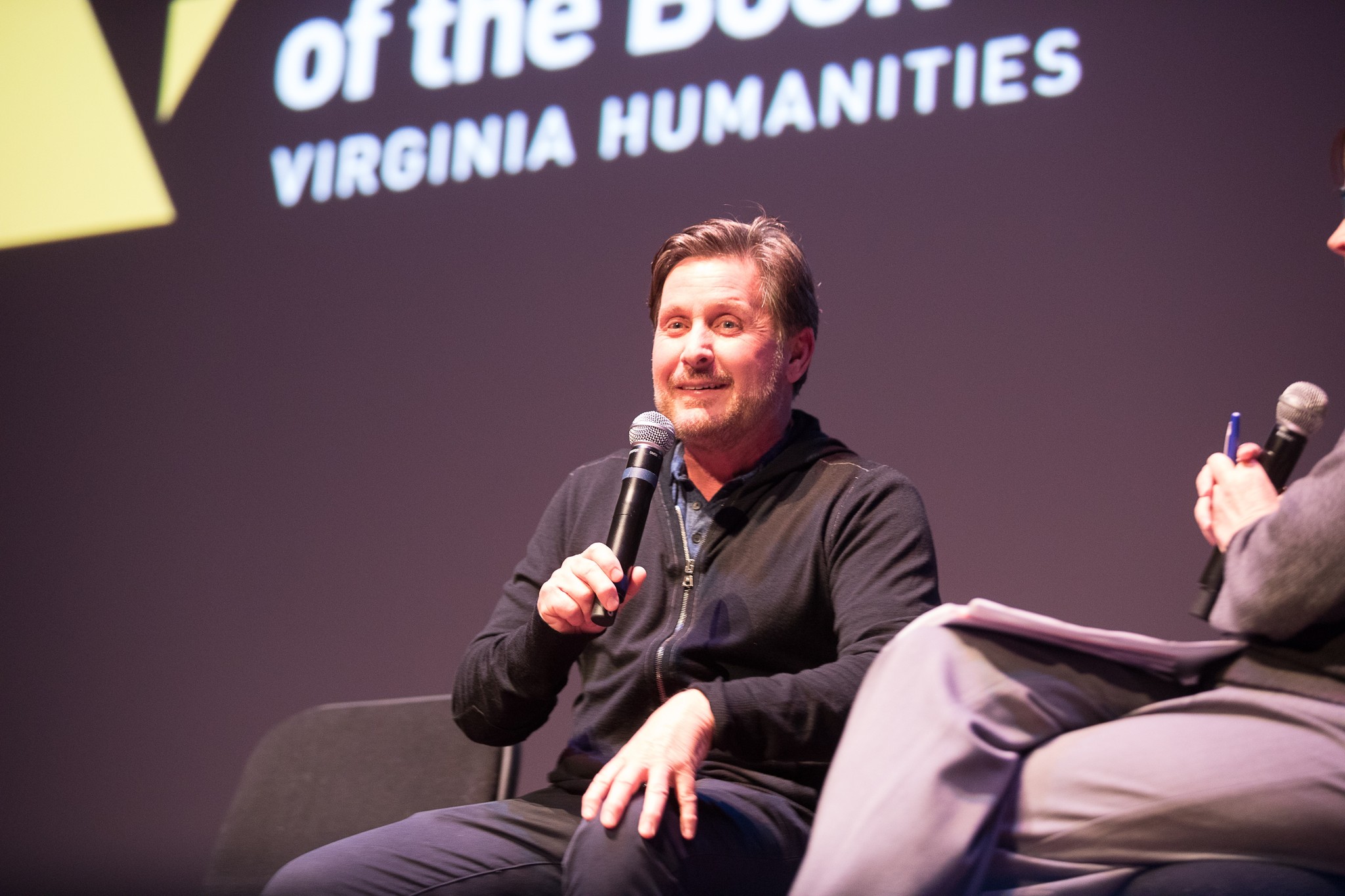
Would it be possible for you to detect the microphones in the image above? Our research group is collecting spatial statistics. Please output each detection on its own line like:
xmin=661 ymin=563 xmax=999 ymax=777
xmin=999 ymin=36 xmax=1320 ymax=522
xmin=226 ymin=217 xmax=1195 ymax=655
xmin=590 ymin=410 xmax=676 ymax=627
xmin=1188 ymin=382 xmax=1328 ymax=620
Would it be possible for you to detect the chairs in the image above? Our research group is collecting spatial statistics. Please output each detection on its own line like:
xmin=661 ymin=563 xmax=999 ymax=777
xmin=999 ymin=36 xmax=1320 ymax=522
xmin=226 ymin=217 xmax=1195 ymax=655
xmin=203 ymin=694 xmax=518 ymax=896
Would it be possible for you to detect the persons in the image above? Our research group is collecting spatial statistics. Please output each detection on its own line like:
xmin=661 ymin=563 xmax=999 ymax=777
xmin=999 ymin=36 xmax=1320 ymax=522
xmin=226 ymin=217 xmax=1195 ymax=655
xmin=260 ymin=216 xmax=945 ymax=896
xmin=786 ymin=223 xmax=1344 ymax=896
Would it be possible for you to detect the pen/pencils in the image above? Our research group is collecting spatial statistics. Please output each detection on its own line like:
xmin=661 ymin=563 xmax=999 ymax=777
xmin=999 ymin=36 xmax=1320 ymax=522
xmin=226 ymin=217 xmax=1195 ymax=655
xmin=1223 ymin=412 xmax=1241 ymax=465
